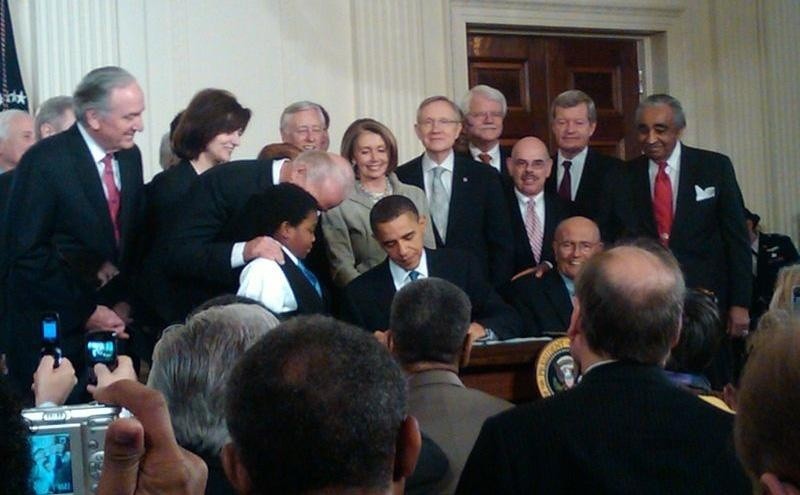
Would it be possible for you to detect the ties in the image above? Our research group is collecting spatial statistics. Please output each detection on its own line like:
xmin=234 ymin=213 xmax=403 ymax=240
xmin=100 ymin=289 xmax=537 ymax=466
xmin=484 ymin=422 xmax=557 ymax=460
xmin=557 ymin=159 xmax=573 ymax=203
xmin=652 ymin=162 xmax=675 ymax=248
xmin=100 ymin=153 xmax=121 ymax=244
xmin=297 ymin=259 xmax=322 ymax=298
xmin=430 ymin=167 xmax=451 ymax=245
xmin=524 ymin=198 xmax=544 ymax=264
xmin=409 ymin=271 xmax=419 ymax=283
xmin=478 ymin=153 xmax=493 ymax=165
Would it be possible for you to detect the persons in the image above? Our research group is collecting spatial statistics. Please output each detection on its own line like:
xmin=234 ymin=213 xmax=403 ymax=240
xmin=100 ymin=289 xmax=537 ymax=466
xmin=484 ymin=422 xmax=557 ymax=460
xmin=87 ymin=355 xmax=138 ymax=401
xmin=664 ymin=287 xmax=736 ymax=415
xmin=383 ymin=278 xmax=516 ymax=495
xmin=0 ymin=67 xmax=143 ymax=405
xmin=461 ymin=84 xmax=511 ymax=172
xmin=395 ymin=97 xmax=516 ymax=289
xmin=146 ymin=87 xmax=251 ymax=240
xmin=35 ymin=98 xmax=79 ymax=139
xmin=505 ymin=137 xmax=578 ymax=264
xmin=0 ymin=108 xmax=36 ymax=173
xmin=320 ymin=118 xmax=437 ymax=289
xmin=221 ymin=314 xmax=421 ymax=495
xmin=236 ymin=182 xmax=333 ymax=316
xmin=319 ymin=105 xmax=330 ymax=152
xmin=145 ymin=304 xmax=280 ymax=457
xmin=452 ymin=106 xmax=473 ymax=162
xmin=92 ymin=381 xmax=207 ymax=495
xmin=512 ymin=94 xmax=751 ymax=339
xmin=32 ymin=355 xmax=78 ymax=407
xmin=517 ymin=217 xmax=604 ymax=338
xmin=733 ymin=323 xmax=800 ymax=495
xmin=548 ymin=90 xmax=630 ymax=225
xmin=151 ymin=151 xmax=355 ymax=323
xmin=455 ymin=240 xmax=755 ymax=495
xmin=745 ymin=205 xmax=800 ymax=314
xmin=280 ymin=102 xmax=330 ymax=153
xmin=344 ymin=195 xmax=523 ymax=347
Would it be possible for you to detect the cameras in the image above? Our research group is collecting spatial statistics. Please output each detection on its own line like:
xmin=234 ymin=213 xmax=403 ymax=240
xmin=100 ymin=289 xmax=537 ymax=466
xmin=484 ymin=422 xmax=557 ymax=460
xmin=18 ymin=401 xmax=121 ymax=494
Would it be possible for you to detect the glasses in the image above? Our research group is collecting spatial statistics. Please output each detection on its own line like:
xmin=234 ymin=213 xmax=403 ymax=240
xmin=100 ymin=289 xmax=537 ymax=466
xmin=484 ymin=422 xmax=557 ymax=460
xmin=469 ymin=110 xmax=504 ymax=119
xmin=421 ymin=118 xmax=458 ymax=127
xmin=555 ymin=118 xmax=586 ymax=128
xmin=557 ymin=237 xmax=599 ymax=252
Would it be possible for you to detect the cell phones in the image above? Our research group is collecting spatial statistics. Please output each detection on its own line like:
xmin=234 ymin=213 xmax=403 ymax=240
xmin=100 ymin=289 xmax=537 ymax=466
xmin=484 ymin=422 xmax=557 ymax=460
xmin=83 ymin=330 xmax=118 ymax=387
xmin=39 ymin=311 xmax=64 ymax=368
